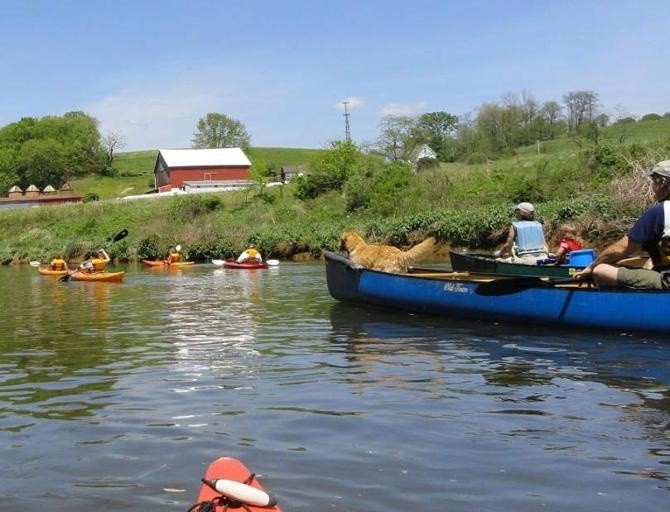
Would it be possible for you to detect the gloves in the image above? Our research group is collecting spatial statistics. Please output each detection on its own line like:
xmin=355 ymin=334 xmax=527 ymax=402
xmin=494 ymin=250 xmax=502 ymax=257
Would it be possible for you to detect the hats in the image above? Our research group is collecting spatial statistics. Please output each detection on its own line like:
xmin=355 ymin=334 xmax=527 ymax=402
xmin=643 ymin=160 xmax=670 ymax=177
xmin=512 ymin=202 xmax=535 ymax=213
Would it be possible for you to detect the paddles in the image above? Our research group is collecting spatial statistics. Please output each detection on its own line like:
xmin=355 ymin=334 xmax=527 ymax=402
xmin=176 ymin=244 xmax=180 ymax=253
xmin=211 ymin=259 xmax=280 ymax=266
xmin=98 ymin=230 xmax=128 ymax=254
xmin=57 ymin=269 xmax=81 ymax=283
xmin=615 ymin=256 xmax=643 ymax=266
xmin=30 ymin=262 xmax=48 ymax=267
xmin=474 ymin=277 xmax=592 ymax=297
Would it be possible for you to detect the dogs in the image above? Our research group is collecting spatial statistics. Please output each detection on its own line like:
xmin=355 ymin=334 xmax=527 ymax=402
xmin=338 ymin=229 xmax=436 ymax=274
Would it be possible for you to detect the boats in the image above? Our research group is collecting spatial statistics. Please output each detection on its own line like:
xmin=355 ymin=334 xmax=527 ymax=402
xmin=67 ymin=270 xmax=125 ymax=286
xmin=190 ymin=457 xmax=283 ymax=512
xmin=141 ymin=258 xmax=195 ymax=267
xmin=37 ymin=260 xmax=106 ymax=275
xmin=446 ymin=245 xmax=597 ymax=279
xmin=210 ymin=256 xmax=273 ymax=269
xmin=323 ymin=248 xmax=670 ymax=333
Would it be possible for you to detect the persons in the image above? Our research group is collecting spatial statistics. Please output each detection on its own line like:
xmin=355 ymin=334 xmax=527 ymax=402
xmin=496 ymin=202 xmax=549 ymax=266
xmin=572 ymin=159 xmax=670 ymax=291
xmin=237 ymin=243 xmax=262 ymax=264
xmin=555 ymin=224 xmax=586 ymax=264
xmin=163 ymin=245 xmax=182 ymax=265
xmin=48 ymin=254 xmax=67 ymax=271
xmin=80 ymin=248 xmax=111 ymax=274
